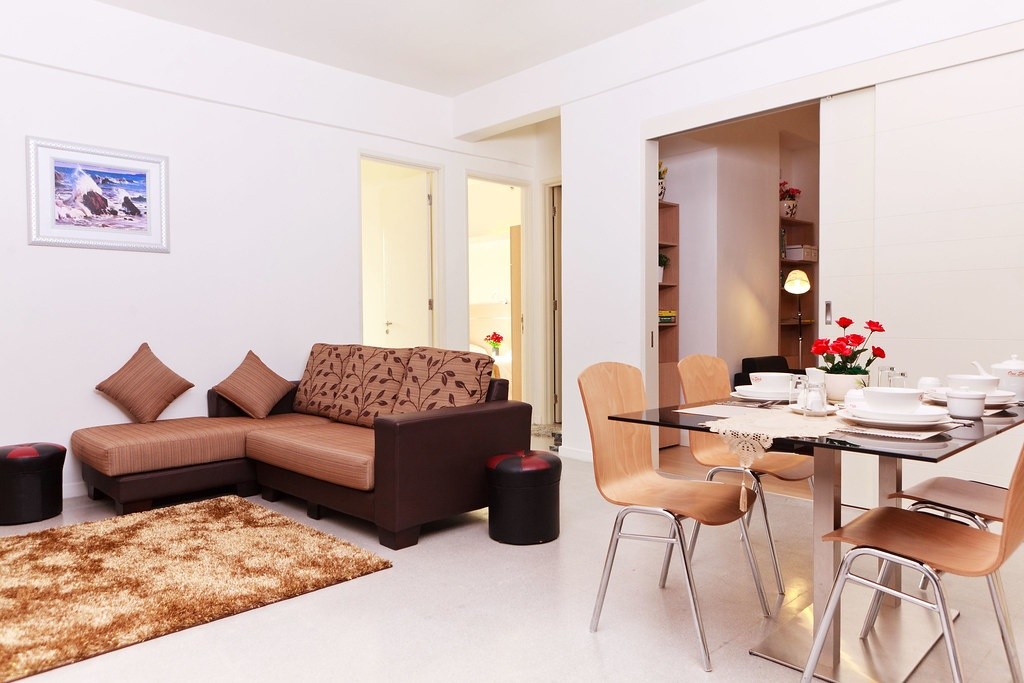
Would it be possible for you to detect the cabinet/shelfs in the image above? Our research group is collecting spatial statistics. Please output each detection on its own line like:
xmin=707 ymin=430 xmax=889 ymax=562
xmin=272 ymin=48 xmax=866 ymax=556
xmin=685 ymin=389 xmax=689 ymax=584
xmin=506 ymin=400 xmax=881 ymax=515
xmin=659 ymin=199 xmax=682 ymax=449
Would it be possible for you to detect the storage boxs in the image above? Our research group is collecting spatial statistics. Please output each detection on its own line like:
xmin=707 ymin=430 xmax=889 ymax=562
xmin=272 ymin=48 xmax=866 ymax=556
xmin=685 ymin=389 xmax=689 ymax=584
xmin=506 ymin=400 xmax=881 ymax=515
xmin=659 ymin=310 xmax=676 ymax=323
xmin=786 ymin=245 xmax=818 ymax=262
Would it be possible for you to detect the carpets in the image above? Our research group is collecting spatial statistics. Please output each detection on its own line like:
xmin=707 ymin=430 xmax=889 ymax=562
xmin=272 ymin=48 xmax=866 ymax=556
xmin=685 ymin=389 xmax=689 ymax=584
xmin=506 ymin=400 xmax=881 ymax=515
xmin=0 ymin=495 xmax=393 ymax=683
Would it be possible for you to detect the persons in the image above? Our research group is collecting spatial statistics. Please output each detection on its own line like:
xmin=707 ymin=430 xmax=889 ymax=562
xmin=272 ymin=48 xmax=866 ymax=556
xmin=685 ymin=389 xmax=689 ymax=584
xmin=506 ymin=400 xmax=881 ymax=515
xmin=122 ymin=196 xmax=141 ymax=215
xmin=469 ymin=333 xmax=493 ymax=355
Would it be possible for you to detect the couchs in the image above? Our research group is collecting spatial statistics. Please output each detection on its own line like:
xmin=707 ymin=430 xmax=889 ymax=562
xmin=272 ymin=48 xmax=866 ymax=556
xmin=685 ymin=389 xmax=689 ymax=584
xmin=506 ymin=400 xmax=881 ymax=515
xmin=72 ymin=344 xmax=533 ymax=550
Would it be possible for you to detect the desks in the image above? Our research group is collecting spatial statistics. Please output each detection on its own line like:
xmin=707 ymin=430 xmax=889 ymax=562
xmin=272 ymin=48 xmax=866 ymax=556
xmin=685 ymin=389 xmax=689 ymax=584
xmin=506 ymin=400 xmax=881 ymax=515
xmin=607 ymin=378 xmax=1024 ymax=683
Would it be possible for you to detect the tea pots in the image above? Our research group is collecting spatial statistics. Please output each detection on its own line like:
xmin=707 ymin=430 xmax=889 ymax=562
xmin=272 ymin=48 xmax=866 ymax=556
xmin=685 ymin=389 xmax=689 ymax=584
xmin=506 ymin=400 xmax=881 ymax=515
xmin=971 ymin=354 xmax=1024 ymax=401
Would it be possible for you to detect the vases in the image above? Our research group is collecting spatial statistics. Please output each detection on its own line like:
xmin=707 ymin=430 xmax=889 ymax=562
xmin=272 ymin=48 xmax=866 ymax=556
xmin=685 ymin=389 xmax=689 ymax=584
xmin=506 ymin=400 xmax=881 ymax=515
xmin=780 ymin=198 xmax=799 ymax=218
xmin=658 ymin=180 xmax=666 ymax=198
xmin=823 ymin=371 xmax=870 ymax=407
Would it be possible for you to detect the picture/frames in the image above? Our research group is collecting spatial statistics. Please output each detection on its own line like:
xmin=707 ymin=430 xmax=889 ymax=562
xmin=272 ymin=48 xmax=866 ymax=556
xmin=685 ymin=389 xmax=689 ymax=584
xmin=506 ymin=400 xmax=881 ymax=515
xmin=25 ymin=136 xmax=170 ymax=254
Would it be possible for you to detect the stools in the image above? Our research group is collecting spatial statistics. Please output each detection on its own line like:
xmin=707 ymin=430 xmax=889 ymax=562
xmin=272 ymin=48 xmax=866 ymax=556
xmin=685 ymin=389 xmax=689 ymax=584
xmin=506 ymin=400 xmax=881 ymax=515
xmin=484 ymin=449 xmax=562 ymax=546
xmin=0 ymin=443 xmax=67 ymax=527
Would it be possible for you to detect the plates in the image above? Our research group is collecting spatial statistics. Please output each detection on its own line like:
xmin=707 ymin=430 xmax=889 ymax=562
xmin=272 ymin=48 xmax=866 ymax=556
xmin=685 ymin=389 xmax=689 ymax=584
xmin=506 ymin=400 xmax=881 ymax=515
xmin=730 ymin=384 xmax=797 ymax=402
xmin=927 ymin=387 xmax=1015 ymax=402
xmin=834 ymin=410 xmax=950 ymax=429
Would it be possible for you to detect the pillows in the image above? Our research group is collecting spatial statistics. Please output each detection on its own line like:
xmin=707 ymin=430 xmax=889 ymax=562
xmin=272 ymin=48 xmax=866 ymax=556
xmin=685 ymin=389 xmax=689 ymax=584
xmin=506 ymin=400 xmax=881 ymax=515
xmin=95 ymin=342 xmax=194 ymax=424
xmin=211 ymin=349 xmax=299 ymax=420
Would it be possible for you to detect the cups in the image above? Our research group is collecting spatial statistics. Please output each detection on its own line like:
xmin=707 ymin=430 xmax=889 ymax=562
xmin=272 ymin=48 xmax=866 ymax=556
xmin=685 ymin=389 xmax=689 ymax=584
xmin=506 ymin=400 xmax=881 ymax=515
xmin=789 ymin=374 xmax=809 ymax=411
xmin=887 ymin=373 xmax=908 ymax=390
xmin=804 ymin=384 xmax=827 ymax=421
xmin=878 ymin=366 xmax=894 ymax=387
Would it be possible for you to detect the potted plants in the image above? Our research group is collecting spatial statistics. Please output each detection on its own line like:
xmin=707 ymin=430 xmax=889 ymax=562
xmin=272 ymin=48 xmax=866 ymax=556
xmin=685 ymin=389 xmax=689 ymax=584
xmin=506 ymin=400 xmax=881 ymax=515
xmin=659 ymin=251 xmax=671 ymax=282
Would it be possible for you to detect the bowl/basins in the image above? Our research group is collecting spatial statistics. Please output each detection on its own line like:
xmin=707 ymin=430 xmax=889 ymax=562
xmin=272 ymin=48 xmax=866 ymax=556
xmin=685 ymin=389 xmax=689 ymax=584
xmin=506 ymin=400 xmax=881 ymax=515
xmin=946 ymin=392 xmax=986 ymax=419
xmin=947 ymin=375 xmax=1000 ymax=392
xmin=749 ymin=373 xmax=792 ymax=390
xmin=864 ymin=386 xmax=924 ymax=413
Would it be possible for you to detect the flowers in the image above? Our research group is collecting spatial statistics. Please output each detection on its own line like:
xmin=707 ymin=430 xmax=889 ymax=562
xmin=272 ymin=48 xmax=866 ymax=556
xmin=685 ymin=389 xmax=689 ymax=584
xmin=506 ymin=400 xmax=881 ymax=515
xmin=779 ymin=180 xmax=802 ymax=202
xmin=810 ymin=317 xmax=886 ymax=373
xmin=659 ymin=159 xmax=669 ymax=178
xmin=484 ymin=333 xmax=504 ymax=353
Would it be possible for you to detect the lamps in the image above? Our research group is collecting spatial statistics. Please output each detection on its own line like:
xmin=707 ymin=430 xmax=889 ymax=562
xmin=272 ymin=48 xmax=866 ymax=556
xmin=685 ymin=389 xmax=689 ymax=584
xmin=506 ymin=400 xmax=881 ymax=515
xmin=783 ymin=269 xmax=812 ymax=368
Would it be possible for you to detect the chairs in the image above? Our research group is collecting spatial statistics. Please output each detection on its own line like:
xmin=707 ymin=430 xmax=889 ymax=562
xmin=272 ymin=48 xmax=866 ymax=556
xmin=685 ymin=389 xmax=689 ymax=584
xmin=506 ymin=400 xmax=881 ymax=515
xmin=676 ymin=351 xmax=816 ymax=596
xmin=799 ymin=444 xmax=1024 ymax=683
xmin=578 ymin=359 xmax=770 ymax=672
